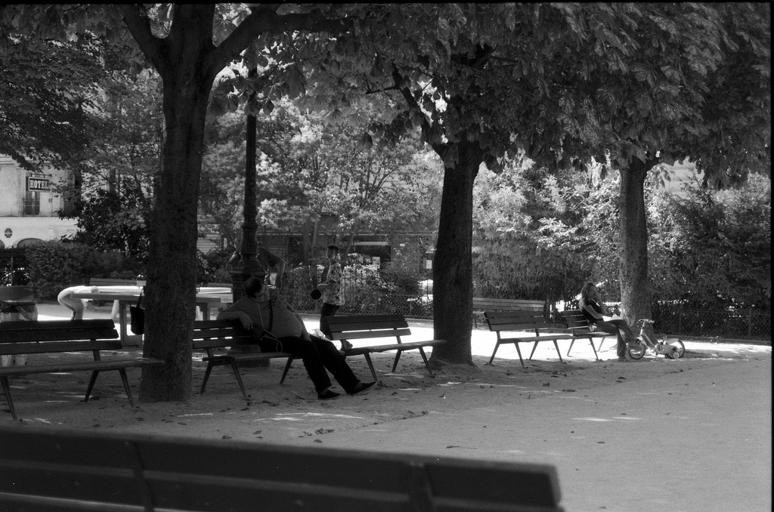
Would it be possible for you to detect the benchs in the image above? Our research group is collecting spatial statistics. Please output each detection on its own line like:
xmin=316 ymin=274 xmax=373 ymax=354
xmin=484 ymin=310 xmax=616 ymax=367
xmin=1 ymin=427 xmax=568 ymax=511
xmin=1 ymin=319 xmax=165 ymax=417
xmin=322 ymin=313 xmax=446 ymax=382
xmin=193 ymin=318 xmax=304 ymax=395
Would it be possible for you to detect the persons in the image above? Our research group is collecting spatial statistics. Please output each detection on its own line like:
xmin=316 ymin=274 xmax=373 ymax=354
xmin=316 ymin=243 xmax=354 ymax=352
xmin=1 ymin=253 xmax=39 ymax=368
xmin=214 ymin=272 xmax=377 ymax=400
xmin=578 ymin=281 xmax=641 ymax=362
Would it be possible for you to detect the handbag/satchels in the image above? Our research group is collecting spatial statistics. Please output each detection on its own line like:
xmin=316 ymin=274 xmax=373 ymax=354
xmin=256 ymin=329 xmax=283 ymax=355
xmin=128 ymin=288 xmax=146 ymax=336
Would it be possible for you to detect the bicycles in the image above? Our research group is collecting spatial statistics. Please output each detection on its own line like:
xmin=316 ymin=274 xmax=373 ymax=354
xmin=628 ymin=318 xmax=685 ymax=360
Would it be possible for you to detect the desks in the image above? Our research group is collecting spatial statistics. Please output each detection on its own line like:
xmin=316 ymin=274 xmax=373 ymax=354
xmin=71 ymin=291 xmax=231 ymax=348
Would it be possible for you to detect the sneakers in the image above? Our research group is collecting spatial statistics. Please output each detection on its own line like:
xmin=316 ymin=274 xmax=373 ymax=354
xmin=348 ymin=381 xmax=377 ymax=397
xmin=316 ymin=390 xmax=342 ymax=401
